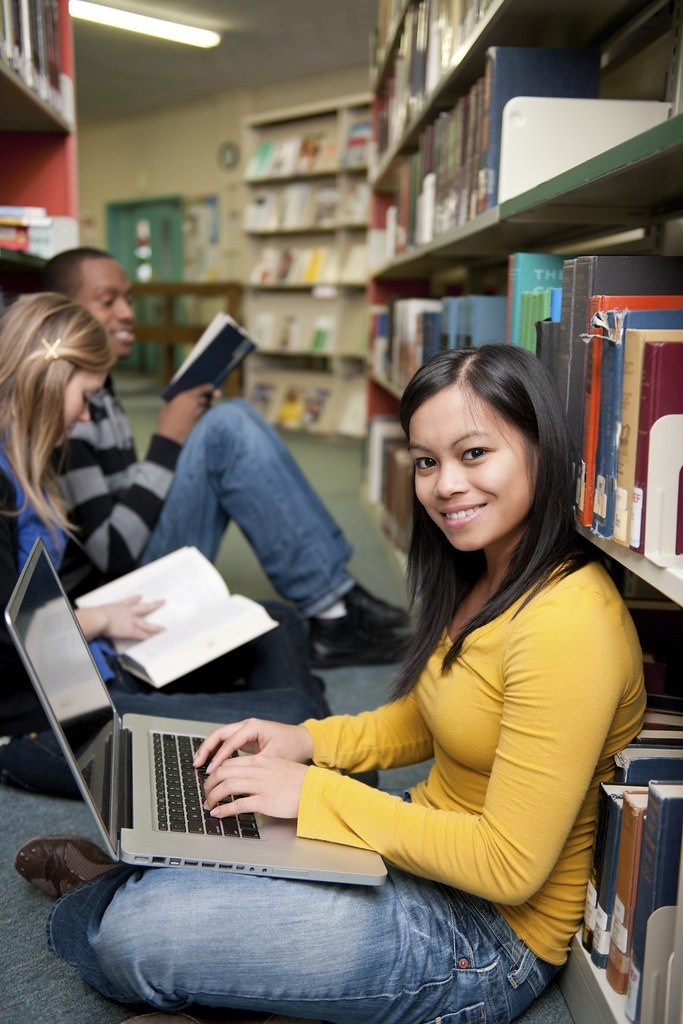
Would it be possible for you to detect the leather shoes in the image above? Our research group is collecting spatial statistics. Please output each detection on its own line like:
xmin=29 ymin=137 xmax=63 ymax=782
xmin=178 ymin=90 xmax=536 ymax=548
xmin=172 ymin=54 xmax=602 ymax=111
xmin=309 ymin=598 xmax=413 ymax=670
xmin=342 ymin=582 xmax=408 ymax=630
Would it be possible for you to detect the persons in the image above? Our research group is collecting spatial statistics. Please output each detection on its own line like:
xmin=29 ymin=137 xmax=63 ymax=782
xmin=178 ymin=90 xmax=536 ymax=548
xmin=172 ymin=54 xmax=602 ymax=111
xmin=0 ymin=292 xmax=382 ymax=807
xmin=33 ymin=247 xmax=413 ymax=668
xmin=15 ymin=342 xmax=650 ymax=1024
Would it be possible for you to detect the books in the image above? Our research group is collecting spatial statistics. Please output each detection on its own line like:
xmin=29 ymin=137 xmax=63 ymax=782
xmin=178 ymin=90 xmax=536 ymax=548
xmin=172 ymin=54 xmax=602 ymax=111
xmin=0 ymin=0 xmax=73 ymax=258
xmin=76 ymin=546 xmax=280 ymax=690
xmin=236 ymin=0 xmax=683 ymax=1024
xmin=158 ymin=312 xmax=254 ymax=403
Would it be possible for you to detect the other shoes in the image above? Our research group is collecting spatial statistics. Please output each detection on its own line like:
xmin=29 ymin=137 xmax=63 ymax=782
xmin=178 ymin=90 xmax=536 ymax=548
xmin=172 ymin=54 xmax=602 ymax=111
xmin=118 ymin=1012 xmax=201 ymax=1024
xmin=14 ymin=834 xmax=122 ymax=900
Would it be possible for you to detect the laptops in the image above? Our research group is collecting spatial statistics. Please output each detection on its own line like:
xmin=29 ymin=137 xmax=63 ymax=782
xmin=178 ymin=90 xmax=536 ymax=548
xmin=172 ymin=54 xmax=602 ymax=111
xmin=6 ymin=536 xmax=387 ymax=886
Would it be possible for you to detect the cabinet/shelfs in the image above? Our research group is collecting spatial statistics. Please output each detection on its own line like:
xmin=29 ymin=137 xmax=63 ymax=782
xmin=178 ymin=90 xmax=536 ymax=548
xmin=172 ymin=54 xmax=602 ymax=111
xmin=238 ymin=91 xmax=368 ymax=445
xmin=363 ymin=0 xmax=683 ymax=1024
xmin=0 ymin=0 xmax=76 ymax=328
